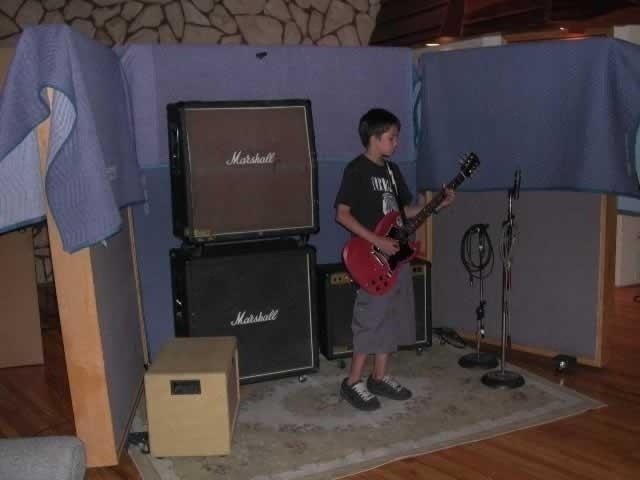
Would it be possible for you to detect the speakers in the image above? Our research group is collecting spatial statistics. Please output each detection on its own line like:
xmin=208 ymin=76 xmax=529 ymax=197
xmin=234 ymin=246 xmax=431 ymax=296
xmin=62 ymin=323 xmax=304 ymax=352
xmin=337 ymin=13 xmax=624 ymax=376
xmin=170 ymin=238 xmax=319 ymax=386
xmin=166 ymin=99 xmax=319 ymax=244
xmin=317 ymin=256 xmax=431 ymax=360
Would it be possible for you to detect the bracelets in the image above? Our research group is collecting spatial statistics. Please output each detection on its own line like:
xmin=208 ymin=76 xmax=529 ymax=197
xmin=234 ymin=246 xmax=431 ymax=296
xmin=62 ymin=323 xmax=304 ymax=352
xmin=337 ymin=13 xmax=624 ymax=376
xmin=433 ymin=208 xmax=439 ymax=215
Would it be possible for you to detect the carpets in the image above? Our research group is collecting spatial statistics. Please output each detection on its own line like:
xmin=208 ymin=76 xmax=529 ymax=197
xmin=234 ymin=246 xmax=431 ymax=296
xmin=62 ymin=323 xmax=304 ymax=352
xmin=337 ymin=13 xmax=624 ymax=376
xmin=127 ymin=333 xmax=608 ymax=480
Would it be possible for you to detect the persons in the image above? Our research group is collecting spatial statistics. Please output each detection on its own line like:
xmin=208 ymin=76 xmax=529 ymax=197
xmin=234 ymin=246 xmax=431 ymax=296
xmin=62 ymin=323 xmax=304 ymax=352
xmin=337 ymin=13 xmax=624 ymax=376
xmin=333 ymin=107 xmax=455 ymax=410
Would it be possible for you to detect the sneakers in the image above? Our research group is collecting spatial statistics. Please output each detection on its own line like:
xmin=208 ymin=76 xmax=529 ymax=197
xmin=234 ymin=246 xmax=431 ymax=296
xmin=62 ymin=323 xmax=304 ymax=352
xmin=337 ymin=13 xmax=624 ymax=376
xmin=367 ymin=374 xmax=412 ymax=400
xmin=340 ymin=377 xmax=380 ymax=410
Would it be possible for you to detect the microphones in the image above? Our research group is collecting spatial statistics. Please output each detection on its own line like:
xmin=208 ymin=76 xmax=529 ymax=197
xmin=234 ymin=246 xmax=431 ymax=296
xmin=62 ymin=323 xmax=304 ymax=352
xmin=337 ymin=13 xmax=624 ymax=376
xmin=514 ymin=169 xmax=521 ymax=200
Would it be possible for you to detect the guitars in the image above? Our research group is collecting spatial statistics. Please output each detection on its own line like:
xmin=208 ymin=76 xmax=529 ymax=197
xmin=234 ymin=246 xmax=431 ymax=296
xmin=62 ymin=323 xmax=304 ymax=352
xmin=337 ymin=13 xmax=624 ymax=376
xmin=340 ymin=152 xmax=480 ymax=297
